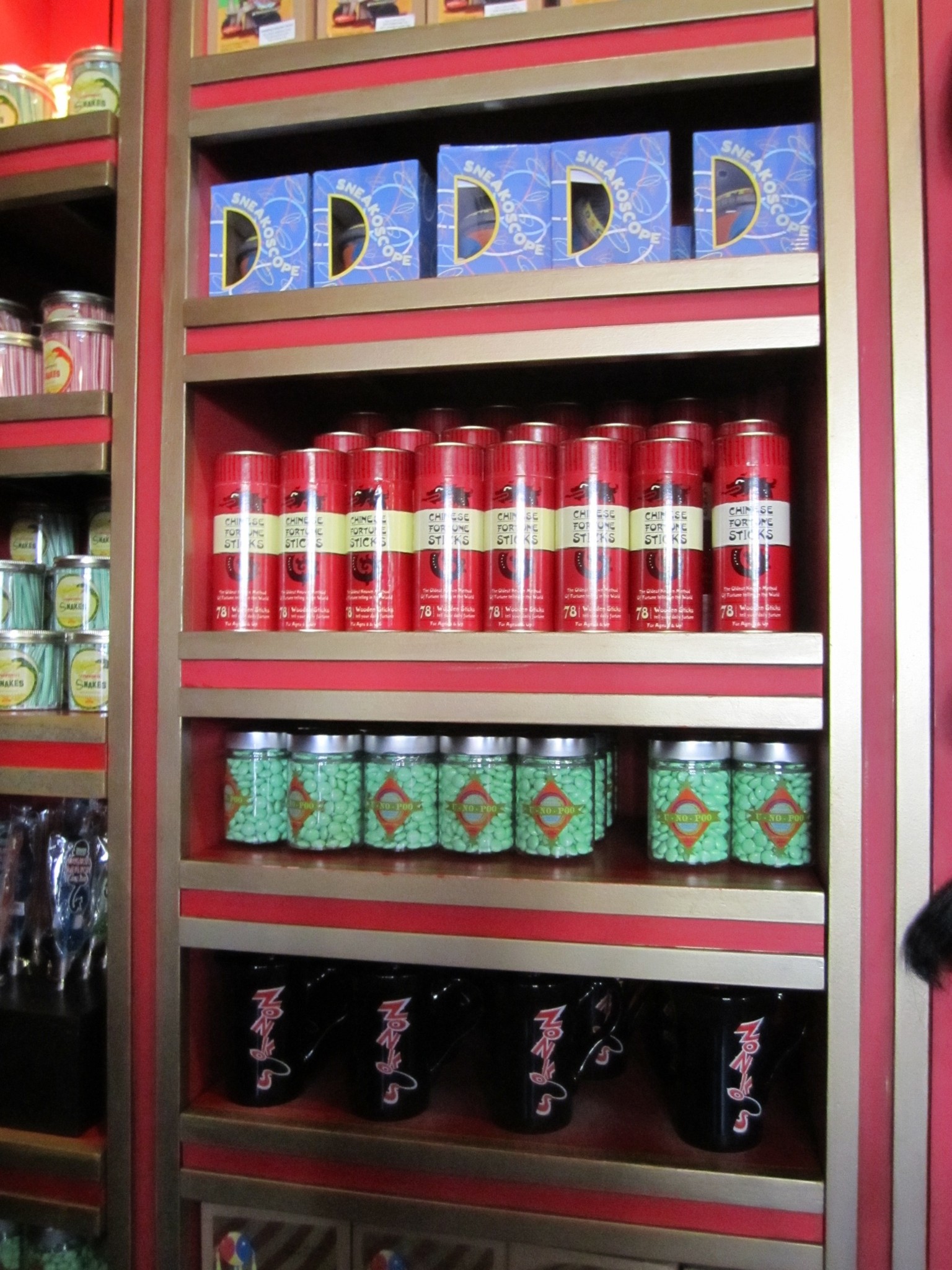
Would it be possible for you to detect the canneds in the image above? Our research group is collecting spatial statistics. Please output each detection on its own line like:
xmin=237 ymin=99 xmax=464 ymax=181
xmin=211 ymin=397 xmax=792 ymax=631
xmin=224 ymin=727 xmax=815 ymax=867
xmin=0 ymin=44 xmax=122 ymax=128
xmin=2 ymin=495 xmax=111 ymax=714
xmin=0 ymin=292 xmax=114 ymax=397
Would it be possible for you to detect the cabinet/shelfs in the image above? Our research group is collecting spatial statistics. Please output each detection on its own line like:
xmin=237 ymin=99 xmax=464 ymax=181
xmin=1 ymin=105 xmax=142 ymax=1270
xmin=172 ymin=0 xmax=862 ymax=1269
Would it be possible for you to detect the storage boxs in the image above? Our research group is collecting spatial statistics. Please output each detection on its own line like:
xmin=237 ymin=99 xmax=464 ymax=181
xmin=437 ymin=141 xmax=552 ymax=275
xmin=552 ymin=128 xmax=675 ymax=270
xmin=693 ymin=122 xmax=820 ymax=261
xmin=206 ymin=169 xmax=311 ymax=298
xmin=313 ymin=158 xmax=437 ymax=287
